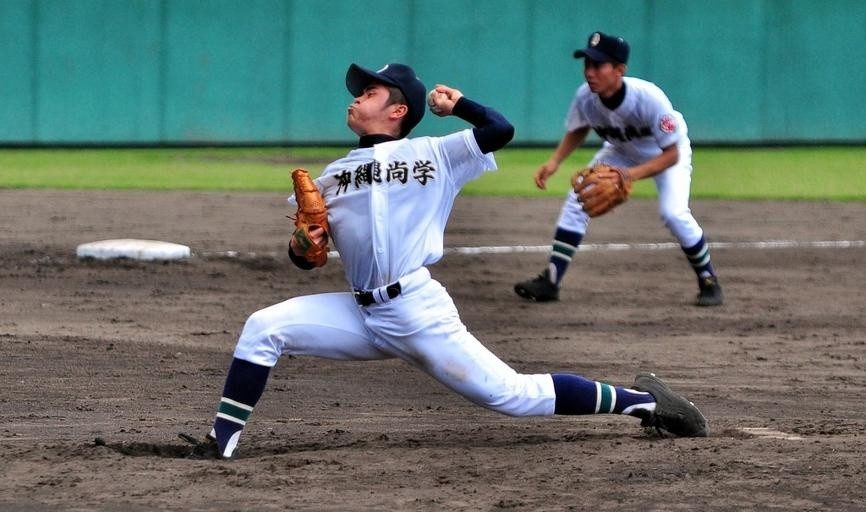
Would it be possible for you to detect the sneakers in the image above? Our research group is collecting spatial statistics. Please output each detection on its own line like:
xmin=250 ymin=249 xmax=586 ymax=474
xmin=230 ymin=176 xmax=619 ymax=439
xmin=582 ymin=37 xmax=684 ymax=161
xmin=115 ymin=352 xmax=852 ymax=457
xmin=635 ymin=373 xmax=713 ymax=439
xmin=179 ymin=434 xmax=233 ymax=460
xmin=696 ymin=276 xmax=724 ymax=309
xmin=512 ymin=270 xmax=561 ymax=304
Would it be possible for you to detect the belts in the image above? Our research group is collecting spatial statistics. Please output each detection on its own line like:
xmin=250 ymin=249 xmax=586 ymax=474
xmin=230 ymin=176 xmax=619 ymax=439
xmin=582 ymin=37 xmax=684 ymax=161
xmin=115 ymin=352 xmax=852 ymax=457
xmin=354 ymin=284 xmax=401 ymax=307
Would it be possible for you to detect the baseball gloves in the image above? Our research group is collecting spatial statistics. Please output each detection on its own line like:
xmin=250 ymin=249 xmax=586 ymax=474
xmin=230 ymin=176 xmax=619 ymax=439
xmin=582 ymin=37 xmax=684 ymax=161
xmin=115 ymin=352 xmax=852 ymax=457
xmin=291 ymin=168 xmax=331 ymax=269
xmin=572 ymin=161 xmax=633 ymax=218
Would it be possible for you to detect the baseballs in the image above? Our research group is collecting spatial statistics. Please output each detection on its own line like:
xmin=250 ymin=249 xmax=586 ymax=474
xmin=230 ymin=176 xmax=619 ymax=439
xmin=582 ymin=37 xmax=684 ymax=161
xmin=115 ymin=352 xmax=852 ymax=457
xmin=427 ymin=90 xmax=449 ymax=113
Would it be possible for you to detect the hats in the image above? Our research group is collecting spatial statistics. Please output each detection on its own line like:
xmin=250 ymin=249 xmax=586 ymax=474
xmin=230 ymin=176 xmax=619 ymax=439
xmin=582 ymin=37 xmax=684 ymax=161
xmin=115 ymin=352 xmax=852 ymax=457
xmin=346 ymin=61 xmax=427 ymax=130
xmin=571 ymin=31 xmax=632 ymax=64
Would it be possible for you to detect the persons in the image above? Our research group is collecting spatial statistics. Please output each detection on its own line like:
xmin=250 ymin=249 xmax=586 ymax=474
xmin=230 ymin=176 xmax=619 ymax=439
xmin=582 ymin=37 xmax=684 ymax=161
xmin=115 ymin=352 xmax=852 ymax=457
xmin=180 ymin=63 xmax=710 ymax=460
xmin=515 ymin=31 xmax=723 ymax=307
